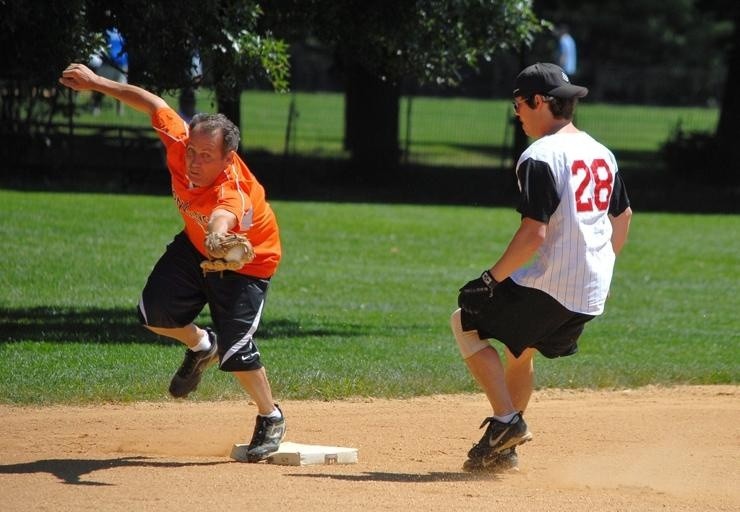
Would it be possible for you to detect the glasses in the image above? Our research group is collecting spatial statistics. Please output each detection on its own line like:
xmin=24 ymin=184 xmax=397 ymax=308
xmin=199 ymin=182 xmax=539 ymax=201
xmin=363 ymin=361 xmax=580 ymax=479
xmin=512 ymin=95 xmax=554 ymax=116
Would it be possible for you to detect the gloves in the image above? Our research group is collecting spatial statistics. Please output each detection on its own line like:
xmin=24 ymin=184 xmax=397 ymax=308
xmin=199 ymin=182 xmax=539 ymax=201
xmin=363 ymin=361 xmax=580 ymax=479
xmin=458 ymin=272 xmax=498 ymax=315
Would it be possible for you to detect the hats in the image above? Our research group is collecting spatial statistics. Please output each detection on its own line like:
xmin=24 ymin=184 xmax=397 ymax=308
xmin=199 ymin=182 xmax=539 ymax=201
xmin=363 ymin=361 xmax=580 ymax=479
xmin=514 ymin=63 xmax=588 ymax=97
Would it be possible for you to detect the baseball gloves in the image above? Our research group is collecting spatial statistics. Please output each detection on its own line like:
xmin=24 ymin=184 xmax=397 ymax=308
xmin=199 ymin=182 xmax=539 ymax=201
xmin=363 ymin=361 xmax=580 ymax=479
xmin=200 ymin=234 xmax=255 ymax=278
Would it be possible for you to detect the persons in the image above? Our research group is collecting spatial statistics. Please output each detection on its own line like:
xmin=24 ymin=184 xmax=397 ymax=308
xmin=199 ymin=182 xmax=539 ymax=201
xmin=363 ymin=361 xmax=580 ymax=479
xmin=552 ymin=22 xmax=578 ymax=79
xmin=59 ymin=60 xmax=285 ymax=462
xmin=86 ymin=28 xmax=129 ymax=120
xmin=448 ymin=62 xmax=635 ymax=474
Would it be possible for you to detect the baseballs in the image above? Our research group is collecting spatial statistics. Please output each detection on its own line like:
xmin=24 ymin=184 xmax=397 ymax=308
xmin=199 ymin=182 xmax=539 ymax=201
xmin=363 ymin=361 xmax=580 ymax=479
xmin=224 ymin=246 xmax=242 ymax=261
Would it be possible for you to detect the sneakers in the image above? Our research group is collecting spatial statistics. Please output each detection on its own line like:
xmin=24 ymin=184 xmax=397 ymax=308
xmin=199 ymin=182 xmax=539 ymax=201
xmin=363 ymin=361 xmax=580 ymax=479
xmin=463 ymin=445 xmax=519 ymax=471
xmin=468 ymin=412 xmax=532 ymax=458
xmin=247 ymin=404 xmax=286 ymax=460
xmin=169 ymin=326 xmax=220 ymax=397
